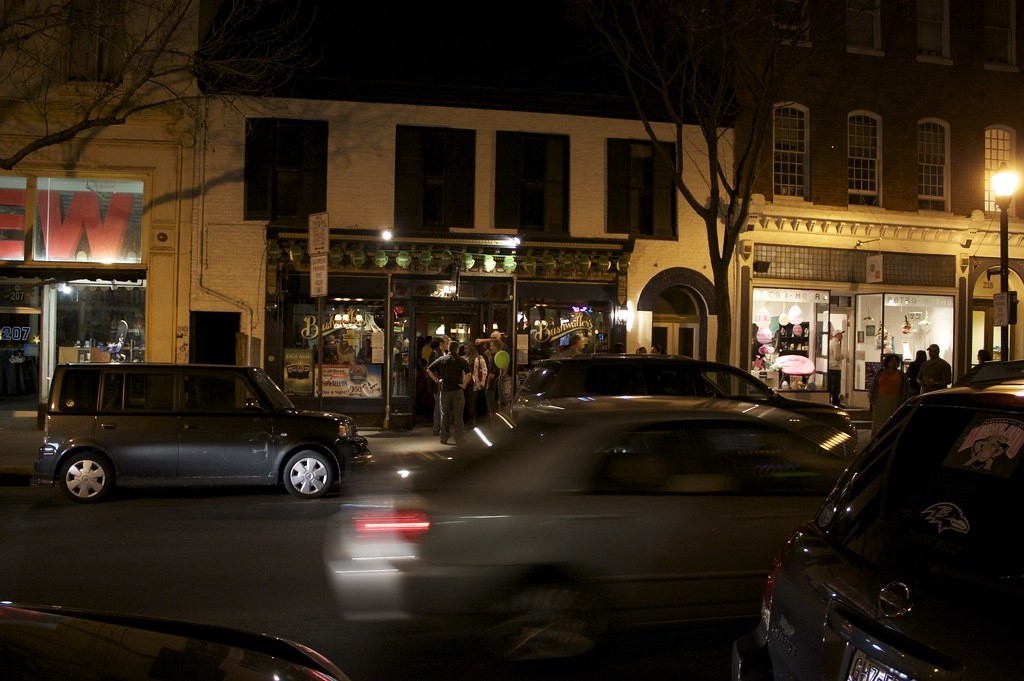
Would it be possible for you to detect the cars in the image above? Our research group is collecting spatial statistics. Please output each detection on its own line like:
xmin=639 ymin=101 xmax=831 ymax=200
xmin=0 ymin=600 xmax=354 ymax=680
xmin=346 ymin=396 xmax=869 ymax=675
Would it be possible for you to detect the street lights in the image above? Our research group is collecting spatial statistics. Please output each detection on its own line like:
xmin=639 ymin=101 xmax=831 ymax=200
xmin=987 ymin=163 xmax=1023 ymax=362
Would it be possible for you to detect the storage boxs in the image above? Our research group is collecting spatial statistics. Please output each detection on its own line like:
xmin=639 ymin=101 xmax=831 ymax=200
xmin=751 ymin=370 xmax=780 ymax=389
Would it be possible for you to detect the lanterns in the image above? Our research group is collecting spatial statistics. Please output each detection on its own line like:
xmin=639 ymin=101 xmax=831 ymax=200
xmin=328 ymin=248 xmax=629 ymax=274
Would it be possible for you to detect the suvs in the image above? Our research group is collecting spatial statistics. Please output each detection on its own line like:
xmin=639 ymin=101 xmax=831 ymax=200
xmin=510 ymin=350 xmax=857 ymax=452
xmin=731 ymin=360 xmax=1024 ymax=680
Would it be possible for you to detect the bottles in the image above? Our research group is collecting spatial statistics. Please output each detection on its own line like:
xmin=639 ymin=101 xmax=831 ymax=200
xmin=75 ymin=341 xmax=81 ymax=348
xmin=84 ymin=341 xmax=90 ymax=361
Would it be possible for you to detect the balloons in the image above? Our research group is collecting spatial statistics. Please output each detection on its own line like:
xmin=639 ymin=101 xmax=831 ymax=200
xmin=495 ymin=351 xmax=510 ymax=370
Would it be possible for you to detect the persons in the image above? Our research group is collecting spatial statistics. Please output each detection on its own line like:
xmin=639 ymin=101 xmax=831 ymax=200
xmin=562 ymin=333 xmax=585 ymax=357
xmin=916 ymin=344 xmax=951 ymax=395
xmin=417 ymin=335 xmax=489 ymax=383
xmin=751 ymin=324 xmax=765 ymax=361
xmin=905 ymin=351 xmax=926 ymax=396
xmin=868 ymin=354 xmax=911 ymax=440
xmin=650 ymin=345 xmax=661 ymax=353
xmin=357 ymin=339 xmax=372 ymax=363
xmin=463 ymin=342 xmax=488 ymax=429
xmin=429 ymin=335 xmax=452 ymax=435
xmin=973 ymin=350 xmax=990 ymax=369
xmin=636 ymin=347 xmax=647 ymax=354
xmin=491 ymin=331 xmax=511 ymax=355
xmin=497 ymin=373 xmax=514 ymax=414
xmin=426 ymin=341 xmax=472 ymax=444
xmin=327 ymin=336 xmax=355 ymax=363
xmin=827 ymin=329 xmax=847 ymax=409
xmin=485 ymin=339 xmax=502 ymax=417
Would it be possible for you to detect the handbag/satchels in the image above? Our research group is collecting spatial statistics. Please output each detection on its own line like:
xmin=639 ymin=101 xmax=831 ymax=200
xmin=478 ymin=356 xmax=483 ymax=381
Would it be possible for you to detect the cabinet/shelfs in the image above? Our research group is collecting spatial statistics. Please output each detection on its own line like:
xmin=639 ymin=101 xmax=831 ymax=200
xmin=778 ymin=321 xmax=831 ymax=388
xmin=122 ymin=329 xmax=144 ymax=363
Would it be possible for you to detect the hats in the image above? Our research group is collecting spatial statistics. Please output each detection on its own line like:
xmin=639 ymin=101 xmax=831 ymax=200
xmin=927 ymin=344 xmax=939 ymax=352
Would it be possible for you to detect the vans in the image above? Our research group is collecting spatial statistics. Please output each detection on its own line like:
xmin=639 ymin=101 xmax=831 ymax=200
xmin=34 ymin=362 xmax=371 ymax=505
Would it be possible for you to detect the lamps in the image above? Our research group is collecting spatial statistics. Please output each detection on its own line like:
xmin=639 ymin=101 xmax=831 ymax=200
xmin=863 ymin=307 xmax=873 ymax=321
xmin=779 ymin=302 xmax=788 ymax=326
xmin=788 ymin=302 xmax=804 ymax=325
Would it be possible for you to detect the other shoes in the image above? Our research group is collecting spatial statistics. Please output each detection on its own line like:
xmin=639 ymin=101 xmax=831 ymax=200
xmin=433 ymin=432 xmax=439 ymax=436
xmin=440 ymin=441 xmax=447 ymax=444
